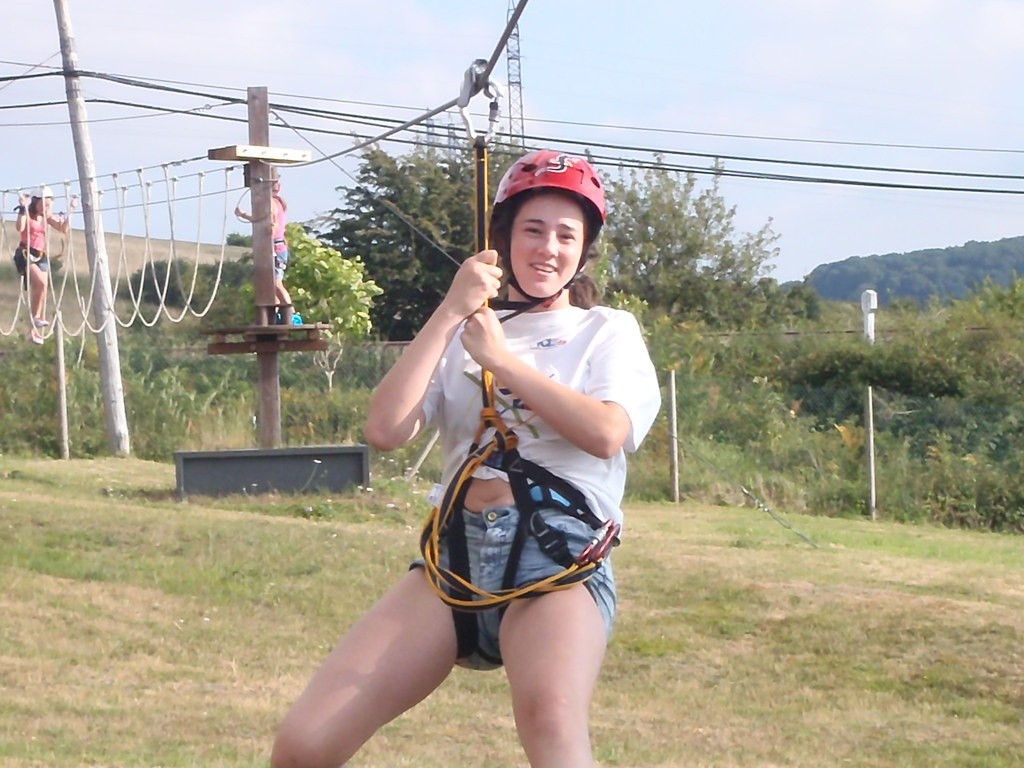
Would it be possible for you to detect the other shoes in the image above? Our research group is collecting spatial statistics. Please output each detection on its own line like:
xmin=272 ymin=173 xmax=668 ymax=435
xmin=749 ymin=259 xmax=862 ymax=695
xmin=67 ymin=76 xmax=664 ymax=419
xmin=31 ymin=331 xmax=45 ymax=345
xmin=31 ymin=320 xmax=47 ymax=327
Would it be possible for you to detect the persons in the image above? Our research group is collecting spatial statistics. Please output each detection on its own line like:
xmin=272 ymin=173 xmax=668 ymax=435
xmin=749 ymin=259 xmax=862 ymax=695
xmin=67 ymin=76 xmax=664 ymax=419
xmin=235 ymin=183 xmax=295 ymax=314
xmin=13 ymin=185 xmax=78 ymax=344
xmin=270 ymin=151 xmax=661 ymax=768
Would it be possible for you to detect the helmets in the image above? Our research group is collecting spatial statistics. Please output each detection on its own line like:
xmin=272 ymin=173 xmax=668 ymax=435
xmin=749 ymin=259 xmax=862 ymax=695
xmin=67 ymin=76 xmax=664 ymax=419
xmin=272 ymin=171 xmax=280 ymax=194
xmin=34 ymin=186 xmax=53 ymax=196
xmin=493 ymin=149 xmax=608 ymax=222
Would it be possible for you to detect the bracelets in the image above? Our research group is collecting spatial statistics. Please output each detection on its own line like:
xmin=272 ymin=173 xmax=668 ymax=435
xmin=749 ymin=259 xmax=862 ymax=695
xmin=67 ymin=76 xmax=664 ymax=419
xmin=243 ymin=213 xmax=246 ymax=217
xmin=71 ymin=204 xmax=74 ymax=208
